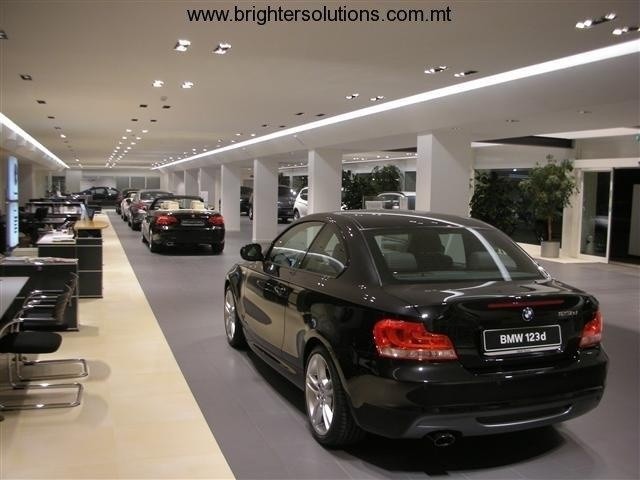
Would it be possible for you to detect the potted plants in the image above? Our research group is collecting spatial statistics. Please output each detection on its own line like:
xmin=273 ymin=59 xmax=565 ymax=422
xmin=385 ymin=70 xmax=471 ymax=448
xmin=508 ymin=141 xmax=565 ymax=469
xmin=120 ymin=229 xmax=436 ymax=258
xmin=518 ymin=150 xmax=581 ymax=259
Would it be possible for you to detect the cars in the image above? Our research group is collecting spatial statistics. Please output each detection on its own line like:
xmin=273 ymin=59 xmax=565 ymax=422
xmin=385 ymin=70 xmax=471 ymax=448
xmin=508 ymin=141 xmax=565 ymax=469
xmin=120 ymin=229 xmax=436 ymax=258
xmin=72 ymin=186 xmax=224 ymax=253
xmin=224 ymin=211 xmax=609 ymax=452
xmin=240 ymin=187 xmax=355 ymax=222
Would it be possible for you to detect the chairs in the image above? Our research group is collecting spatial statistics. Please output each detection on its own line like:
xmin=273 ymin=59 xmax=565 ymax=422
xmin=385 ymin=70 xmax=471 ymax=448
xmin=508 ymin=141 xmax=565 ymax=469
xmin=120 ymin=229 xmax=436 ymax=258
xmin=0 ymin=269 xmax=89 ymax=411
xmin=161 ymin=199 xmax=205 ymax=210
xmin=383 ymin=231 xmax=518 ymax=278
